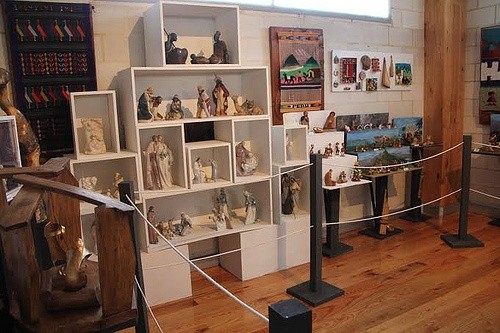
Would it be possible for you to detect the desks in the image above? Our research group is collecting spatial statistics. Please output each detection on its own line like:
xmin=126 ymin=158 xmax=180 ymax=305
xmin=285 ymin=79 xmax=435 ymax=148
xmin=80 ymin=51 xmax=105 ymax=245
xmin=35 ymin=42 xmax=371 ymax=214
xmin=358 ymin=165 xmax=424 ymax=241
xmin=323 ymin=180 xmax=375 ymax=259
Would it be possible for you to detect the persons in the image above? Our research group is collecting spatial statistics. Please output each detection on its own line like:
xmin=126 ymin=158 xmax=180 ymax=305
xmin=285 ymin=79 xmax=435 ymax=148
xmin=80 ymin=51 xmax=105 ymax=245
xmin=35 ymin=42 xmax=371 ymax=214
xmin=324 ymin=111 xmax=336 ymax=128
xmin=217 ymin=188 xmax=233 ymax=220
xmin=112 ymin=171 xmax=124 ymax=201
xmin=193 ymin=156 xmax=208 ymax=185
xmin=165 ymin=94 xmax=185 ymax=120
xmin=281 ymin=172 xmax=302 ymax=214
xmin=242 ymin=190 xmax=257 ymax=225
xmin=157 ymin=211 xmax=194 ymax=237
xmin=300 ymin=108 xmax=309 ymax=128
xmin=212 ymin=77 xmax=230 ymax=116
xmin=142 ymin=134 xmax=177 ymax=193
xmin=196 ymin=85 xmax=213 ymax=119
xmin=138 ymin=87 xmax=163 ymax=122
xmin=165 ymin=32 xmax=177 ymax=58
xmin=208 ymin=30 xmax=229 ymax=63
xmin=236 ymin=140 xmax=253 ymax=176
xmin=324 ymin=162 xmax=362 ymax=186
xmin=147 ymin=205 xmax=160 ymax=244
xmin=310 ymin=139 xmax=346 ymax=159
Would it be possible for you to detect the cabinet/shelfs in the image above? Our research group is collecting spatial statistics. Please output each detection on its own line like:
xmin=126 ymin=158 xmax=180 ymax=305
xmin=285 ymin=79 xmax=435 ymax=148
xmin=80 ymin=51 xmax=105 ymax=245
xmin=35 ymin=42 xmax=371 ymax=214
xmin=63 ymin=0 xmax=323 ymax=315
